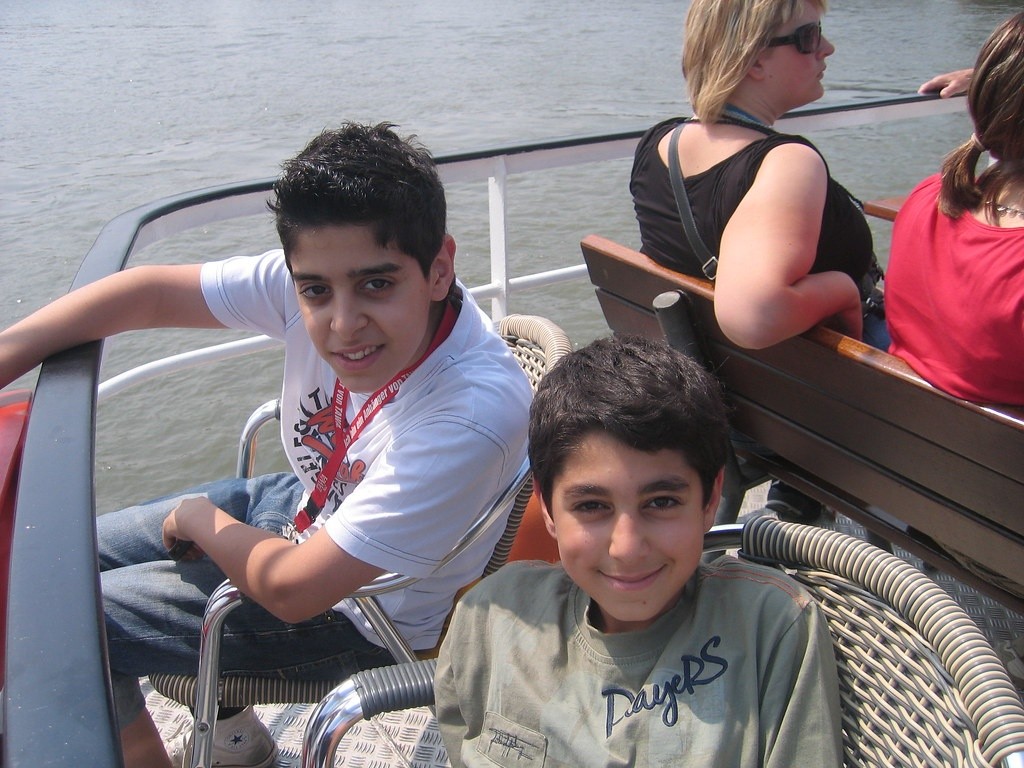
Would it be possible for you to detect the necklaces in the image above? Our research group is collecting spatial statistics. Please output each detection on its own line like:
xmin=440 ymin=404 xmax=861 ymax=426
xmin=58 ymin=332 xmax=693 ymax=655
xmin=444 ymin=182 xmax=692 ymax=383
xmin=983 ymin=199 xmax=1024 ymax=218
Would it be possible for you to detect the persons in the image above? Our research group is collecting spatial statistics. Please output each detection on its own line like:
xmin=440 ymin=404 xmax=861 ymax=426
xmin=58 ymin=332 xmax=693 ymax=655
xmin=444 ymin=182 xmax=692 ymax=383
xmin=883 ymin=12 xmax=1024 ymax=419
xmin=630 ymin=0 xmax=891 ymax=354
xmin=0 ymin=121 xmax=533 ymax=768
xmin=435 ymin=334 xmax=845 ymax=768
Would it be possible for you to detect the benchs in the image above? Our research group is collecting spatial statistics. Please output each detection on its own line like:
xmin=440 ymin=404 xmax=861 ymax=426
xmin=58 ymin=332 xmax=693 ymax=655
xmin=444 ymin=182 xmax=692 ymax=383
xmin=580 ymin=234 xmax=1024 ymax=696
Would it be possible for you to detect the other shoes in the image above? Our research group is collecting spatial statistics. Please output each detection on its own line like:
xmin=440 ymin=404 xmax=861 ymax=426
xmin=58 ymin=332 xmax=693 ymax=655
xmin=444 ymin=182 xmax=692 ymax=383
xmin=765 ymin=478 xmax=821 ymax=521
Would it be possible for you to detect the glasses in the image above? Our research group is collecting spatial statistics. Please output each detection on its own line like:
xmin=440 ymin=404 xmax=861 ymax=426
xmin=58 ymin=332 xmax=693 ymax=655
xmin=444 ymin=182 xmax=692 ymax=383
xmin=768 ymin=21 xmax=821 ymax=53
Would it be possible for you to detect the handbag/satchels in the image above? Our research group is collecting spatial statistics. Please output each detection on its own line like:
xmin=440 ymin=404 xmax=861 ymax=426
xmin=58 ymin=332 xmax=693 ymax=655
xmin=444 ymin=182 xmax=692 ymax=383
xmin=865 ymin=288 xmax=886 ymax=320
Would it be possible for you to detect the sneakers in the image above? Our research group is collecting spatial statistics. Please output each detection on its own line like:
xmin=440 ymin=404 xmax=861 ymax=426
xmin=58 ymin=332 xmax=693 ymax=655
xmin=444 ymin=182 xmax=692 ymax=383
xmin=164 ymin=703 xmax=279 ymax=768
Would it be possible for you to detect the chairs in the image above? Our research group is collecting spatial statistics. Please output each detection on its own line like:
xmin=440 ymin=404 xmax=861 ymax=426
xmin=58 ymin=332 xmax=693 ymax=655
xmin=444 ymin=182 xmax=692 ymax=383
xmin=302 ymin=515 xmax=1024 ymax=768
xmin=149 ymin=311 xmax=573 ymax=768
